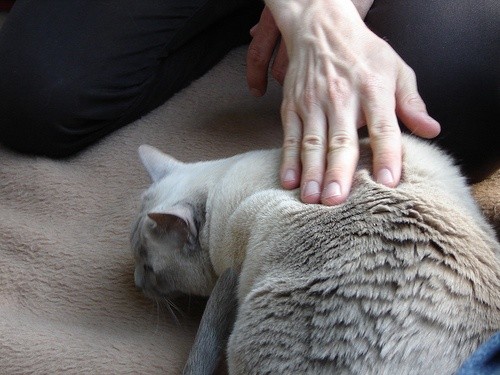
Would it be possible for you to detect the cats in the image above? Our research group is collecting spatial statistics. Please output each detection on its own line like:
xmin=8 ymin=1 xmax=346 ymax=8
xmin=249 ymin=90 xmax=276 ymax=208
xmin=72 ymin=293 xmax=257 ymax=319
xmin=130 ymin=142 xmax=500 ymax=375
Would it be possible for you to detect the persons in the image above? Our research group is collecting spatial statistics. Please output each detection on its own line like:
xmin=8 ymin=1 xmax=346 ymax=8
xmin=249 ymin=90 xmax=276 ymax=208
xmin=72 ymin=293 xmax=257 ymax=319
xmin=0 ymin=0 xmax=499 ymax=207
